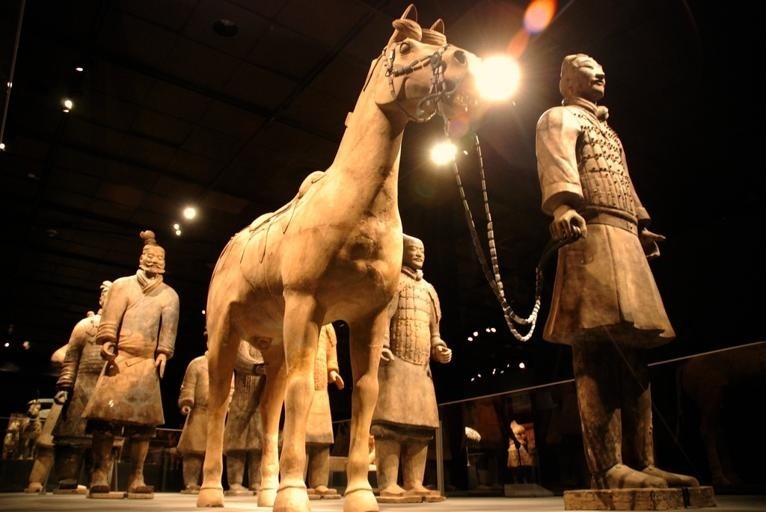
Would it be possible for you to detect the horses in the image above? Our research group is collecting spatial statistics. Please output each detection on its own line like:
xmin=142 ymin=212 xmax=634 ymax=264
xmin=196 ymin=4 xmax=479 ymax=512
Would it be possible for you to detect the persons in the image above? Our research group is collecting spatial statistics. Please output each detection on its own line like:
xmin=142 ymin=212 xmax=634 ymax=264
xmin=370 ymin=230 xmax=453 ymax=496
xmin=533 ymin=52 xmax=704 ymax=490
xmin=51 ymin=278 xmax=112 ymax=490
xmin=79 ymin=231 xmax=181 ymax=493
xmin=223 ymin=339 xmax=267 ymax=494
xmin=26 ymin=308 xmax=95 ymax=497
xmin=176 ymin=339 xmax=235 ymax=492
xmin=304 ymin=321 xmax=346 ymax=494
